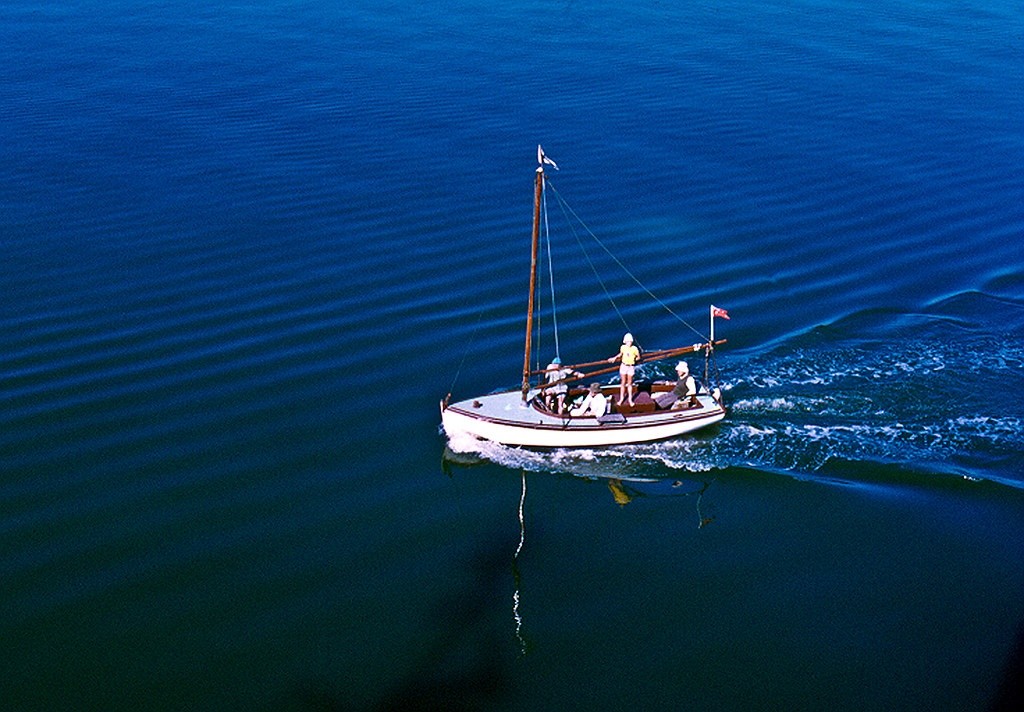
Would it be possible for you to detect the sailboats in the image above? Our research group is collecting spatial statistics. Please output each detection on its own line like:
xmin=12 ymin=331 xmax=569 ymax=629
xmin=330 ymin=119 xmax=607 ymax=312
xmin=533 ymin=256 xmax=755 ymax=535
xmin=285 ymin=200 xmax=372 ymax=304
xmin=436 ymin=146 xmax=732 ymax=445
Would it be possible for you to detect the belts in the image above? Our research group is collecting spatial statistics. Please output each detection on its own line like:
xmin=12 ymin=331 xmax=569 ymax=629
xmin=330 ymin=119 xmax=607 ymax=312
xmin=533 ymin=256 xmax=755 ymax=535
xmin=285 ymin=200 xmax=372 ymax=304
xmin=622 ymin=362 xmax=635 ymax=366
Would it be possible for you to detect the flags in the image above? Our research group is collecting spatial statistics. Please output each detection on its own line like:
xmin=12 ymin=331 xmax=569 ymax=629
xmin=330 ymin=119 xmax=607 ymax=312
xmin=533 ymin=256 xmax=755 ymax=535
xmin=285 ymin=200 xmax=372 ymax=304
xmin=713 ymin=306 xmax=730 ymax=320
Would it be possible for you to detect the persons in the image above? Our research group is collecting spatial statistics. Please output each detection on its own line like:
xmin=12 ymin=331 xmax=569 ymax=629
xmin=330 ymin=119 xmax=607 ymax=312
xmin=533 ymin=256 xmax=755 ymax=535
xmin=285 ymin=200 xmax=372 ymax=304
xmin=671 ymin=362 xmax=696 ymax=409
xmin=608 ymin=333 xmax=640 ymax=406
xmin=545 ymin=357 xmax=585 ymax=414
xmin=609 ymin=478 xmax=633 ymax=508
xmin=585 ymin=383 xmax=607 ymax=417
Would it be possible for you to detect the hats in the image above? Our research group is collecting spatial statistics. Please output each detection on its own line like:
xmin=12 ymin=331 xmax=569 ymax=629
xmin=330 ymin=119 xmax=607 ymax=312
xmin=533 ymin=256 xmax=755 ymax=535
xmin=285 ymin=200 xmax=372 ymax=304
xmin=623 ymin=333 xmax=633 ymax=344
xmin=588 ymin=383 xmax=602 ymax=392
xmin=552 ymin=357 xmax=561 ymax=365
xmin=675 ymin=362 xmax=689 ymax=373
